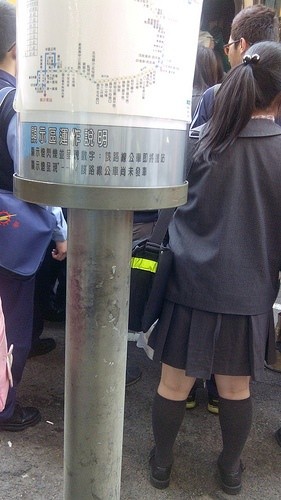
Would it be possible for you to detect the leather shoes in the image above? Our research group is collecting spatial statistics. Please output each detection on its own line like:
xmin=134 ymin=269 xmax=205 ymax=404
xmin=147 ymin=445 xmax=171 ymax=489
xmin=0 ymin=404 xmax=41 ymax=431
xmin=218 ymin=450 xmax=246 ymax=494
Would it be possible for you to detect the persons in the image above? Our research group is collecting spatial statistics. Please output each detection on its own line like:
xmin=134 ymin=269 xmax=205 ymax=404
xmin=145 ymin=40 xmax=280 ymax=494
xmin=0 ymin=0 xmax=281 ymax=432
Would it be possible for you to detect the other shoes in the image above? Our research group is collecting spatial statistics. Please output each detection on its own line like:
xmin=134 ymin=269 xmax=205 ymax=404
xmin=126 ymin=366 xmax=142 ymax=386
xmin=186 ymin=392 xmax=197 ymax=409
xmin=208 ymin=396 xmax=220 ymax=414
xmin=28 ymin=337 xmax=55 ymax=359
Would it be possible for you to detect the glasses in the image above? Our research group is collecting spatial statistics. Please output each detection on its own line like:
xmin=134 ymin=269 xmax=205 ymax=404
xmin=223 ymin=38 xmax=253 ymax=55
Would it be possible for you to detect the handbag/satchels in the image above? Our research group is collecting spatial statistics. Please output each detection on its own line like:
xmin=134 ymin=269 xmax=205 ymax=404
xmin=129 ymin=237 xmax=172 ymax=333
xmin=0 ymin=189 xmax=57 ymax=279
